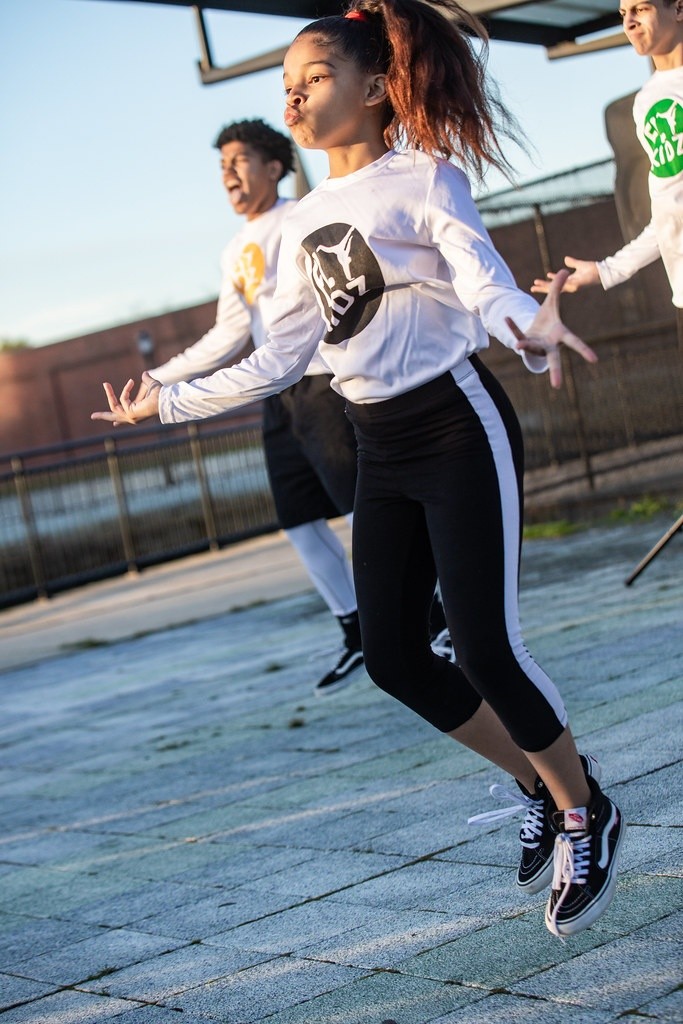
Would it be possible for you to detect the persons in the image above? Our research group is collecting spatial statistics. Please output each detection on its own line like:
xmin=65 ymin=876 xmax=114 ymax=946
xmin=88 ymin=0 xmax=624 ymax=942
xmin=530 ymin=1 xmax=683 ymax=358
xmin=134 ymin=120 xmax=455 ymax=700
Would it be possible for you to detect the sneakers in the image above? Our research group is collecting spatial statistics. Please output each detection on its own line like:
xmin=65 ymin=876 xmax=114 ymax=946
xmin=314 ymin=635 xmax=364 ymax=694
xmin=428 ymin=626 xmax=456 ymax=663
xmin=543 ymin=775 xmax=625 ymax=939
xmin=467 ymin=752 xmax=602 ymax=893
